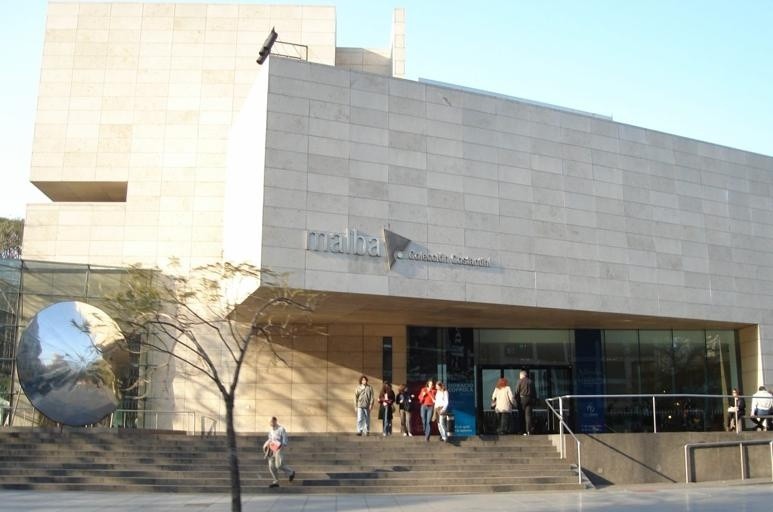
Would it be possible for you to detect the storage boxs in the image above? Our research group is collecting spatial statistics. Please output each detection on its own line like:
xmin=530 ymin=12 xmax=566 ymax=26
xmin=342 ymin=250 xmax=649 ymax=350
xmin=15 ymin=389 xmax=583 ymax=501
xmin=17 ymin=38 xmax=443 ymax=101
xmin=734 ymin=414 xmax=772 ymax=432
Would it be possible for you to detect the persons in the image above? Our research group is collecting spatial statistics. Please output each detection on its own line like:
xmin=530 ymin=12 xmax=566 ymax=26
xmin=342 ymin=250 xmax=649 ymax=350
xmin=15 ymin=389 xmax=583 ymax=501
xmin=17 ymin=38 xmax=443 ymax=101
xmin=418 ymin=377 xmax=436 ymax=441
xmin=516 ymin=369 xmax=535 ymax=436
xmin=354 ymin=376 xmax=373 ymax=436
xmin=491 ymin=378 xmax=518 ymax=434
xmin=396 ymin=384 xmax=415 ymax=436
xmin=378 ymin=381 xmax=395 ymax=436
xmin=262 ymin=417 xmax=295 ymax=487
xmin=434 ymin=381 xmax=450 ymax=442
xmin=726 ymin=388 xmax=746 ymax=431
xmin=751 ymin=386 xmax=773 ymax=430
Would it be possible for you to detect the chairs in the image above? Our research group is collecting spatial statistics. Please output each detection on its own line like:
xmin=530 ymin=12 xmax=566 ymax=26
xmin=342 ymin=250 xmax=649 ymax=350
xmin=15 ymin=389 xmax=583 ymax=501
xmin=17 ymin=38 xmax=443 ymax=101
xmin=269 ymin=484 xmax=279 ymax=488
xmin=289 ymin=470 xmax=295 ymax=481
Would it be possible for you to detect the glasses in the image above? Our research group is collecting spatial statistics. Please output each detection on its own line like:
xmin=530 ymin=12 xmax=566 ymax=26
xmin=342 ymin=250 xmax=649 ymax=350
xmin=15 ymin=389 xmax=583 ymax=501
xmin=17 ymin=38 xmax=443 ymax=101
xmin=255 ymin=29 xmax=309 ymax=64
